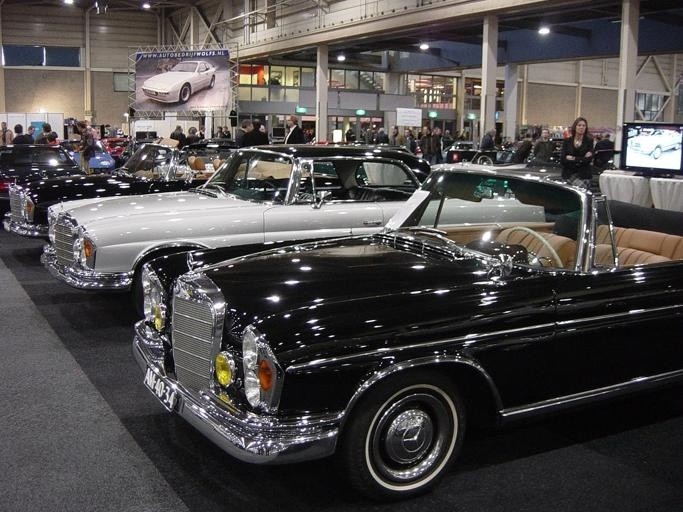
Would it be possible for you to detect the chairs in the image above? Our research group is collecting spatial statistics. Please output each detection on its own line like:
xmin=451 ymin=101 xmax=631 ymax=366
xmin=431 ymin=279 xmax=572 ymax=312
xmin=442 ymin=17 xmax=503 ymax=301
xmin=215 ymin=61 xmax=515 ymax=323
xmin=488 ymin=214 xmax=682 ymax=282
xmin=186 ymin=155 xmax=207 ymax=184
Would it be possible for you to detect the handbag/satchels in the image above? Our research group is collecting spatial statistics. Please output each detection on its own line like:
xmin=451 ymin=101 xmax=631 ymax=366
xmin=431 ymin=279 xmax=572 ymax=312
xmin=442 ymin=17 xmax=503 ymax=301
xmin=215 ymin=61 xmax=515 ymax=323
xmin=83 ymin=145 xmax=95 ymax=161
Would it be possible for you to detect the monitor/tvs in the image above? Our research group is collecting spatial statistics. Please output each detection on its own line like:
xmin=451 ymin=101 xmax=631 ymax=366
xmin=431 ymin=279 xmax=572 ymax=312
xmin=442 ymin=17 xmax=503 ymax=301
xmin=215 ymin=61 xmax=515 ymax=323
xmin=272 ymin=127 xmax=286 ymax=140
xmin=619 ymin=122 xmax=683 ymax=177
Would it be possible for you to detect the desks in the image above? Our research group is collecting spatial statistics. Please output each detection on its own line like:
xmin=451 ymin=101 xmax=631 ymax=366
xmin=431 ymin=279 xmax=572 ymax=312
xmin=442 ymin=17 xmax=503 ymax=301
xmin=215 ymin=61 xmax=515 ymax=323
xmin=598 ymin=165 xmax=682 ymax=219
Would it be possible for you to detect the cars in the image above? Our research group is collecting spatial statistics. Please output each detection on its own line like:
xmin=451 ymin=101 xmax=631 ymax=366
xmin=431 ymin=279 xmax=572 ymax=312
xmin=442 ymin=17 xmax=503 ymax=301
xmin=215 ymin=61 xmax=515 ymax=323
xmin=39 ymin=144 xmax=546 ymax=319
xmin=140 ymin=60 xmax=217 ymax=105
xmin=625 ymin=129 xmax=681 ymax=160
xmin=131 ymin=162 xmax=682 ymax=502
